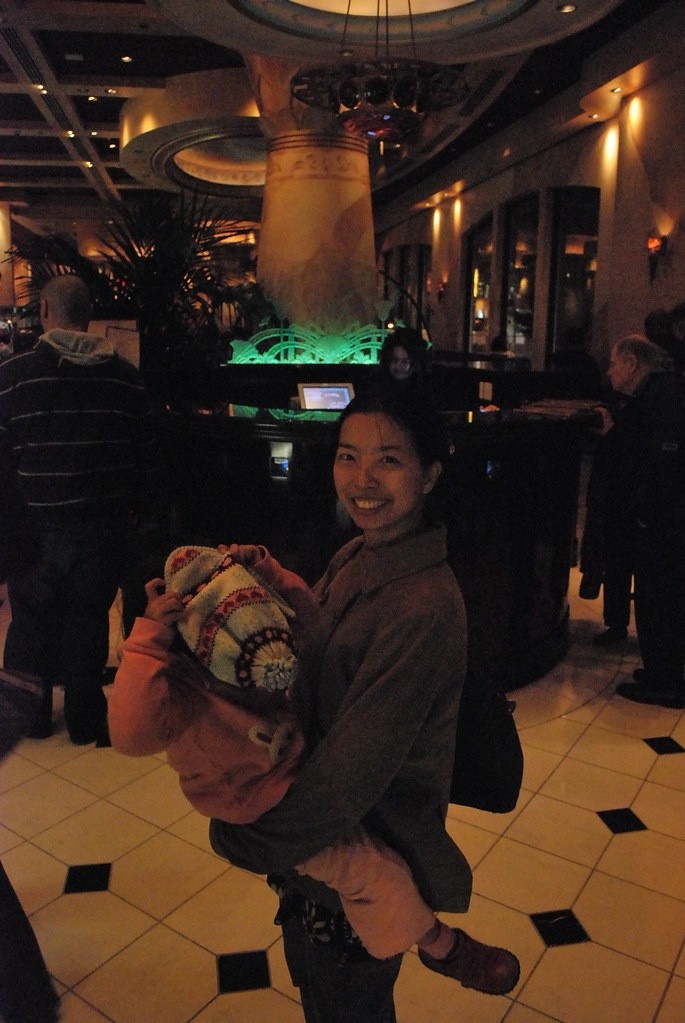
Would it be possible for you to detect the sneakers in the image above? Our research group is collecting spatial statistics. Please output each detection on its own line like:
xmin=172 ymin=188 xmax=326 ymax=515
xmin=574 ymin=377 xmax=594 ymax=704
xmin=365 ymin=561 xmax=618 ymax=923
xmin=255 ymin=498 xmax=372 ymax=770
xmin=418 ymin=928 xmax=521 ymax=995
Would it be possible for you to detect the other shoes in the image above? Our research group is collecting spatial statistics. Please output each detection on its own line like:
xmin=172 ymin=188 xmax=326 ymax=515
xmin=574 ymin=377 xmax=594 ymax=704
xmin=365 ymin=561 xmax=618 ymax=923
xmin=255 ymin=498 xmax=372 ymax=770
xmin=633 ymin=669 xmax=657 ymax=684
xmin=586 ymin=626 xmax=628 ymax=642
xmin=617 ymin=683 xmax=684 ymax=709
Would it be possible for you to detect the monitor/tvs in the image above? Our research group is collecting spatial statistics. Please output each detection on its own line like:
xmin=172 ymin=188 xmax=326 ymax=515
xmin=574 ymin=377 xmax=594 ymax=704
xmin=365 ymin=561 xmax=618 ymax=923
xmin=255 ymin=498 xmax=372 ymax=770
xmin=296 ymin=382 xmax=356 ymax=410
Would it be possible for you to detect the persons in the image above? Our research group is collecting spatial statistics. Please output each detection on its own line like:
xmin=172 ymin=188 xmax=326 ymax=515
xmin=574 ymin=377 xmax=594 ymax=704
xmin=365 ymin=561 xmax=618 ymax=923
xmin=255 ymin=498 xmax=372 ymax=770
xmin=207 ymin=392 xmax=473 ymax=1023
xmin=104 ymin=544 xmax=522 ymax=994
xmin=0 ymin=858 xmax=61 ymax=1023
xmin=580 ymin=334 xmax=685 ymax=711
xmin=340 ymin=329 xmax=436 ymax=431
xmin=0 ymin=276 xmax=172 ymax=749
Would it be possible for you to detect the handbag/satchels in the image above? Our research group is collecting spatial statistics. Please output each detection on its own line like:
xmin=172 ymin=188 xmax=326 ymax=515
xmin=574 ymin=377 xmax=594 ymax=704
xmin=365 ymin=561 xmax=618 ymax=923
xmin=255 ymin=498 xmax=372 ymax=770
xmin=452 ymin=645 xmax=524 ymax=815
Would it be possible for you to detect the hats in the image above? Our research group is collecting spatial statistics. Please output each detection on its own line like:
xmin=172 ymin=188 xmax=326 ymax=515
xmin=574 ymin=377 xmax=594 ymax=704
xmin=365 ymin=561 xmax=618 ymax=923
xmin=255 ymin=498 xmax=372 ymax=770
xmin=165 ymin=546 xmax=297 ymax=692
